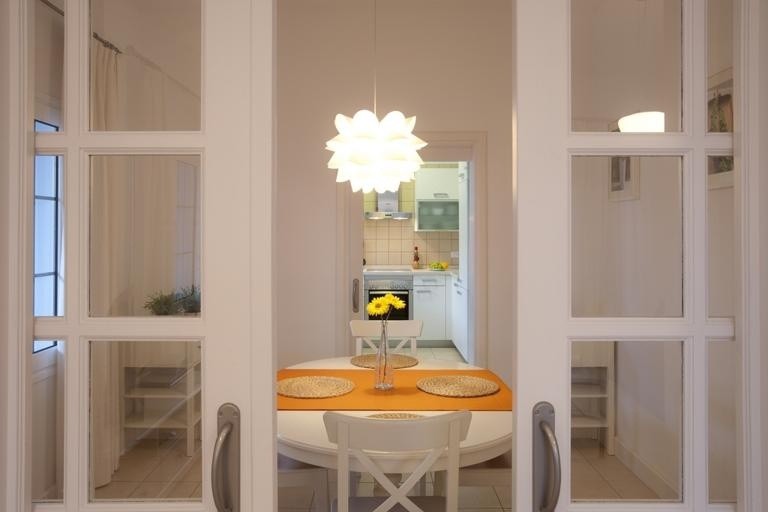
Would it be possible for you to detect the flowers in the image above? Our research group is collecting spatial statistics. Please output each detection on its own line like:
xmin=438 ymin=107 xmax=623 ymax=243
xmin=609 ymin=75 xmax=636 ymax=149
xmin=366 ymin=294 xmax=407 ymax=389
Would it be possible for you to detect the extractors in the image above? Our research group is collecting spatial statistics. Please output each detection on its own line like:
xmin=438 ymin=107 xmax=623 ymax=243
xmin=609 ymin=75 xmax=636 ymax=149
xmin=367 ymin=191 xmax=413 ymax=221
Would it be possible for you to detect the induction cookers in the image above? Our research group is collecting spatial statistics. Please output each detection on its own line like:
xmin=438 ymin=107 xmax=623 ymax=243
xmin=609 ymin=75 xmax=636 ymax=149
xmin=364 ymin=265 xmax=412 ymax=274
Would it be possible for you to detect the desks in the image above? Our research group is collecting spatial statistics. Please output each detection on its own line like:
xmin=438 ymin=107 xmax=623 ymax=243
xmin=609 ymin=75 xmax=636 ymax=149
xmin=278 ymin=354 xmax=513 ymax=512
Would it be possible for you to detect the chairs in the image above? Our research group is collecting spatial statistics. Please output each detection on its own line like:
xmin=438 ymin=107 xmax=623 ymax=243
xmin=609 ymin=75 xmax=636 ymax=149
xmin=323 ymin=409 xmax=472 ymax=512
xmin=350 ymin=319 xmax=424 ymax=357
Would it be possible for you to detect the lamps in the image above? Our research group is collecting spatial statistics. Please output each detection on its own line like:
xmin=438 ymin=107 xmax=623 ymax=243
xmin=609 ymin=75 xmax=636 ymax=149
xmin=323 ymin=0 xmax=429 ymax=196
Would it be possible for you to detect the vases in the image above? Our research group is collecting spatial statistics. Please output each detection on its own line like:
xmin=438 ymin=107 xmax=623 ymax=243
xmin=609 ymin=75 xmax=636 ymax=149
xmin=375 ymin=322 xmax=395 ymax=389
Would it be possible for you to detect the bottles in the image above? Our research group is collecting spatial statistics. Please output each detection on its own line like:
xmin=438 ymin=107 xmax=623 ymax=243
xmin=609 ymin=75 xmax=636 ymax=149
xmin=412 ymin=246 xmax=420 ymax=269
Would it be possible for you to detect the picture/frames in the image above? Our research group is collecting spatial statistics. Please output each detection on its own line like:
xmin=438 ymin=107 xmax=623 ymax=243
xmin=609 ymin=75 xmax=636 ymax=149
xmin=607 ymin=122 xmax=641 ymax=202
xmin=705 ymin=66 xmax=738 ymax=189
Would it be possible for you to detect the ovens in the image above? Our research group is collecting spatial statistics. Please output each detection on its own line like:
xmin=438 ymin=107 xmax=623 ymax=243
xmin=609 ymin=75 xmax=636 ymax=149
xmin=365 ymin=273 xmax=413 ymax=320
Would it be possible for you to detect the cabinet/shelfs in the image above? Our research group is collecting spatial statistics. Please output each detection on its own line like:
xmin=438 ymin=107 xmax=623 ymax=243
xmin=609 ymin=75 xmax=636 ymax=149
xmin=111 ymin=342 xmax=200 ymax=458
xmin=414 ymin=276 xmax=471 ymax=363
xmin=571 ymin=341 xmax=616 ymax=456
xmin=414 ymin=163 xmax=459 ymax=233
